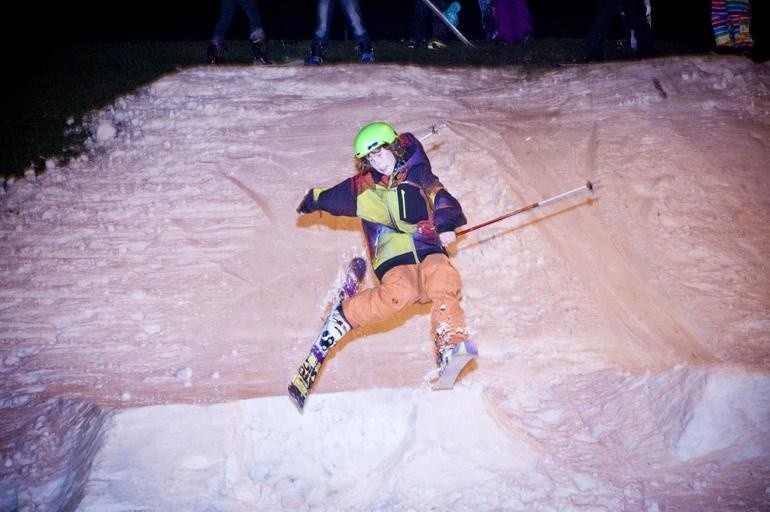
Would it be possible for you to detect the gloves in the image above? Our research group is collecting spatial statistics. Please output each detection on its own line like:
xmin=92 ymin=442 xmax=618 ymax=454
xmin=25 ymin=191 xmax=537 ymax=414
xmin=296 ymin=189 xmax=318 ymax=214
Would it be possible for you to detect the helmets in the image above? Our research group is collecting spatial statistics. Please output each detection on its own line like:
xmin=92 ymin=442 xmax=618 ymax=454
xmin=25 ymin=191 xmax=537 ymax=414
xmin=353 ymin=122 xmax=401 ymax=159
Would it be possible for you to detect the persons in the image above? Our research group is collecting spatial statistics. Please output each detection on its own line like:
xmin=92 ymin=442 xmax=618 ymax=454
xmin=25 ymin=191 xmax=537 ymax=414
xmin=204 ymin=1 xmax=269 ymax=67
xmin=405 ymin=1 xmax=454 ymax=50
xmin=616 ymin=1 xmax=655 ymax=60
xmin=707 ymin=1 xmax=757 ymax=58
xmin=293 ymin=121 xmax=480 ymax=380
xmin=476 ymin=0 xmax=539 ymax=68
xmin=308 ymin=1 xmax=377 ymax=65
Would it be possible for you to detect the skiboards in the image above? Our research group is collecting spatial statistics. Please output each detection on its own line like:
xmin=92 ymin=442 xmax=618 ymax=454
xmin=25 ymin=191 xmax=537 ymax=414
xmin=288 ymin=258 xmax=477 ymax=408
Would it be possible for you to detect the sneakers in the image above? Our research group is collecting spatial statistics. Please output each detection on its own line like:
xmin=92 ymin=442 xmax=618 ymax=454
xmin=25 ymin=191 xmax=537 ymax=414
xmin=441 ymin=344 xmax=457 ymax=367
xmin=328 ymin=308 xmax=351 ymax=341
